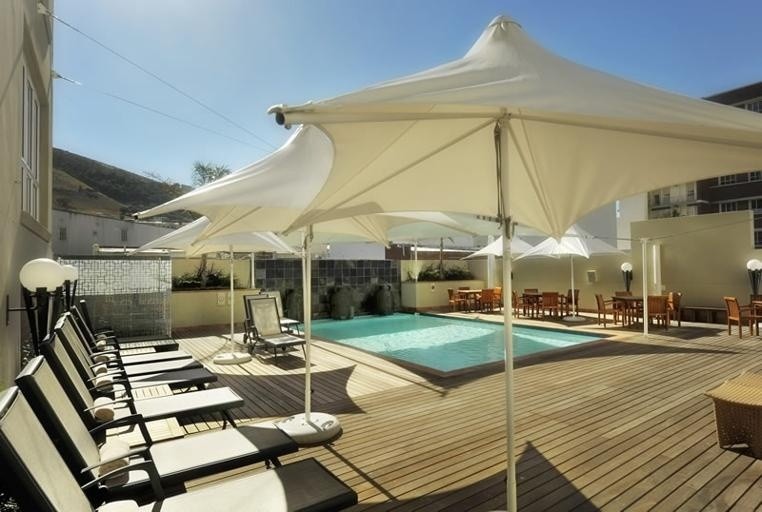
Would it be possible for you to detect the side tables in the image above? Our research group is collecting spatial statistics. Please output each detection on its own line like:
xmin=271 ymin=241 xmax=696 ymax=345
xmin=105 ymin=418 xmax=183 ymax=449
xmin=114 ymin=384 xmax=172 ymax=400
xmin=119 ymin=346 xmax=156 ymax=357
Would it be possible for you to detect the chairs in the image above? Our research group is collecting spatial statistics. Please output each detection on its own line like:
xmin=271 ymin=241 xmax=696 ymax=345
xmin=537 ymin=292 xmax=559 ymax=318
xmin=616 ymin=292 xmax=642 ymax=324
xmin=80 ymin=300 xmax=169 ymax=343
xmin=41 ymin=331 xmax=245 ymax=442
xmin=648 ymin=295 xmax=670 ymax=330
xmin=448 ymin=288 xmax=461 ymax=312
xmin=596 ymin=294 xmax=625 ymax=328
xmin=479 ymin=289 xmax=494 ymax=312
xmin=63 ymin=311 xmax=192 ymax=367
xmin=14 ymin=356 xmax=298 ymax=503
xmin=71 ymin=305 xmax=178 ymax=353
xmin=54 ymin=323 xmax=217 ymax=398
xmin=512 ymin=290 xmax=529 ymax=318
xmin=487 ymin=287 xmax=502 ymax=311
xmin=57 ymin=316 xmax=203 ymax=378
xmin=725 ymin=297 xmax=762 ymax=339
xmin=248 ymin=297 xmax=306 ymax=365
xmin=0 ymin=386 xmax=357 ymax=511
xmin=657 ymin=293 xmax=682 ymax=328
xmin=459 ymin=287 xmax=474 ymax=310
xmin=243 ymin=294 xmax=300 ymax=343
xmin=523 ymin=289 xmax=539 ymax=315
xmin=566 ymin=289 xmax=580 ymax=316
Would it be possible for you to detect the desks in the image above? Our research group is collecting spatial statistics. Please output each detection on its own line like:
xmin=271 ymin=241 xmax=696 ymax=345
xmin=705 ymin=370 xmax=762 ymax=459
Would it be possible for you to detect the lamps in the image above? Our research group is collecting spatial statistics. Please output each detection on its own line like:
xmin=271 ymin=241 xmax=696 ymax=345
xmin=61 ymin=266 xmax=79 ymax=310
xmin=621 ymin=262 xmax=633 ymax=291
xmin=747 ymin=259 xmax=762 ymax=295
xmin=6 ymin=259 xmax=65 ymax=356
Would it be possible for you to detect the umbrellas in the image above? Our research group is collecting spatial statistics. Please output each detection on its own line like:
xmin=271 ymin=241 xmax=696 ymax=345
xmin=266 ymin=14 xmax=761 ymax=510
xmin=458 ymin=231 xmax=561 ymax=264
xmin=124 ymin=213 xmax=302 ymax=354
xmin=143 ymin=125 xmax=482 ymax=415
xmin=512 ymin=222 xmax=627 ymax=316
